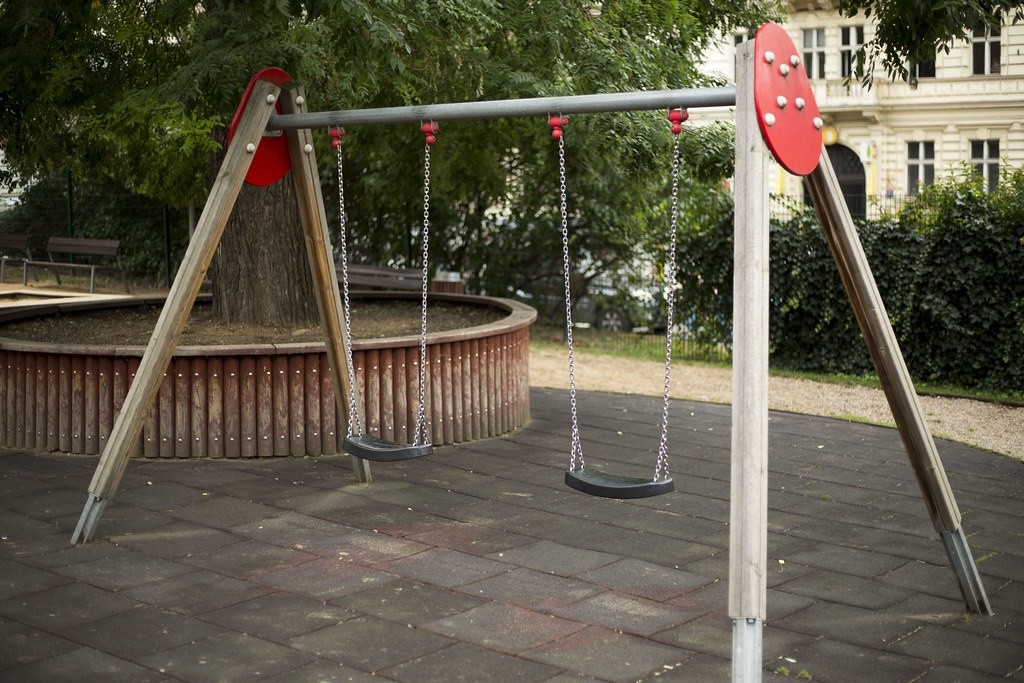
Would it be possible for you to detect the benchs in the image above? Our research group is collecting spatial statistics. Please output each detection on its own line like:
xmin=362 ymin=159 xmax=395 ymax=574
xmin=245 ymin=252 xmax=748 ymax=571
xmin=337 ymin=263 xmax=424 ymax=292
xmin=0 ymin=234 xmax=129 ymax=296
